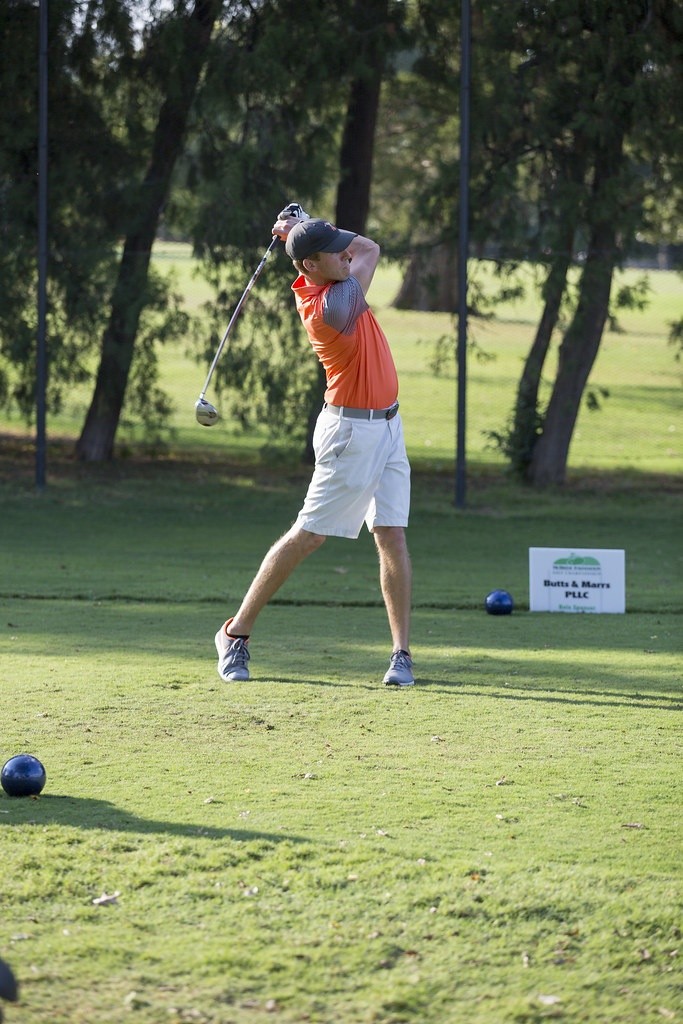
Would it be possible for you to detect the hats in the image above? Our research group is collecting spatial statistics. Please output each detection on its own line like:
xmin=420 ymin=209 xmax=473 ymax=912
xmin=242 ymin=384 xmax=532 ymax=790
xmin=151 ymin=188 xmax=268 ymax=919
xmin=283 ymin=217 xmax=359 ymax=258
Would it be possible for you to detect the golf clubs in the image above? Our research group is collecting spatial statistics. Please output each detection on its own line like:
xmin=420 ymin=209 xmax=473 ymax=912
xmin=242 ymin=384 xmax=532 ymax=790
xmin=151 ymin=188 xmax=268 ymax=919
xmin=193 ymin=234 xmax=281 ymax=427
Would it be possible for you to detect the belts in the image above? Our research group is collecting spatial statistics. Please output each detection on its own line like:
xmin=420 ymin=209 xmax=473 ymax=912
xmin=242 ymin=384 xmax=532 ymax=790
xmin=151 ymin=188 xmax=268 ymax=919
xmin=321 ymin=401 xmax=401 ymax=422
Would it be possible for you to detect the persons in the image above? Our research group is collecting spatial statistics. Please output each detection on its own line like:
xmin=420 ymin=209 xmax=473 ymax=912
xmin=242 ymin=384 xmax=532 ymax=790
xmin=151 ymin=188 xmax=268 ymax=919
xmin=215 ymin=204 xmax=414 ymax=686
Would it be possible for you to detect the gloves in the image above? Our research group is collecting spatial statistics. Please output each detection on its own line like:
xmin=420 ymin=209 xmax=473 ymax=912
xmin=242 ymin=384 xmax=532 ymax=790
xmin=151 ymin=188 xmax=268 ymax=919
xmin=277 ymin=201 xmax=311 ymax=224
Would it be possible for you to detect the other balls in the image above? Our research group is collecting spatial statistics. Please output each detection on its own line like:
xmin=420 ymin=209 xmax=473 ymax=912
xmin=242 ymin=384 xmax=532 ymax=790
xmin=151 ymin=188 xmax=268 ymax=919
xmin=1 ymin=754 xmax=46 ymax=797
xmin=485 ymin=589 xmax=514 ymax=616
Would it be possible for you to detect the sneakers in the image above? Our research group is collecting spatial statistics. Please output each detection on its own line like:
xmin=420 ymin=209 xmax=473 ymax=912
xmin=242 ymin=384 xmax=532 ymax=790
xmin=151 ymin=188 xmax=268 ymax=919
xmin=381 ymin=652 xmax=415 ymax=688
xmin=214 ymin=619 xmax=249 ymax=685
